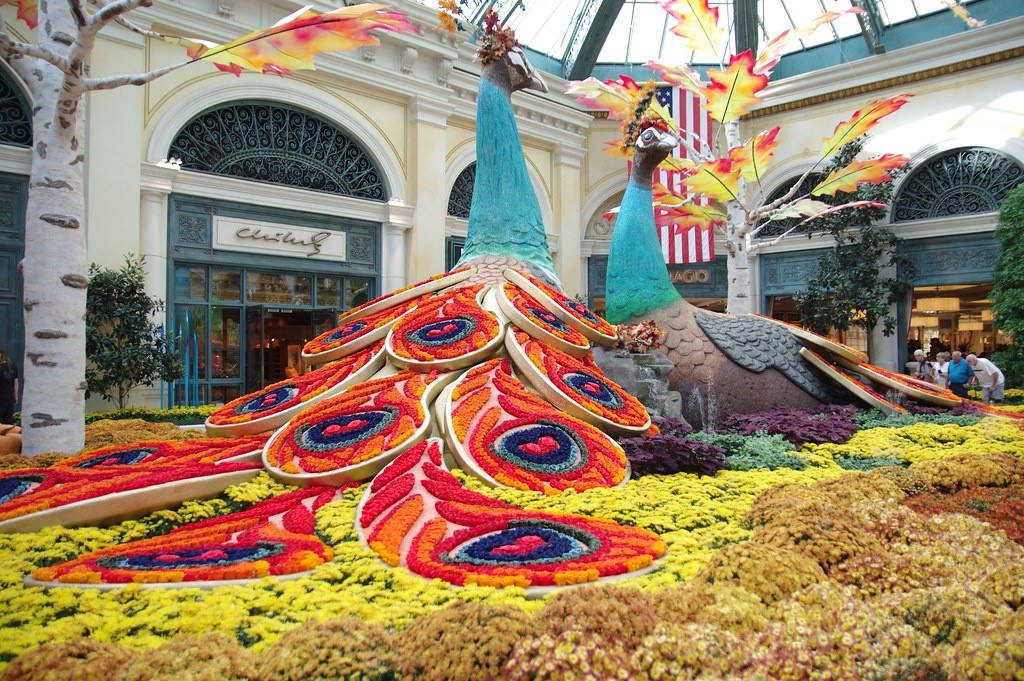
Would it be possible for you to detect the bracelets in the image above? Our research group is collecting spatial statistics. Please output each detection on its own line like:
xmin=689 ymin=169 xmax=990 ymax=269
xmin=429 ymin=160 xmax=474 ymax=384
xmin=967 ymin=382 xmax=970 ymax=386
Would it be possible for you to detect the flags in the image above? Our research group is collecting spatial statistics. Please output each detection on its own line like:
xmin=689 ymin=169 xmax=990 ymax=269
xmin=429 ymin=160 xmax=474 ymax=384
xmin=625 ymin=82 xmax=714 ymax=265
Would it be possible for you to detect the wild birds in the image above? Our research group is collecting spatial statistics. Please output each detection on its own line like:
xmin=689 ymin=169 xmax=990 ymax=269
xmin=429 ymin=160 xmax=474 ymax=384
xmin=1 ymin=0 xmax=1023 ymax=579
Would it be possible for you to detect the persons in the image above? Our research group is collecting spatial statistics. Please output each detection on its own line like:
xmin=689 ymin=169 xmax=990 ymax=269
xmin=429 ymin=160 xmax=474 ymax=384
xmin=912 ymin=349 xmax=934 ymax=384
xmin=966 ymin=353 xmax=1005 ymax=404
xmin=929 ymin=352 xmax=950 ymax=388
xmin=944 ymin=351 xmax=951 ymax=362
xmin=947 ymin=350 xmax=974 ymax=399
xmin=0 ymin=345 xmax=19 ymax=425
